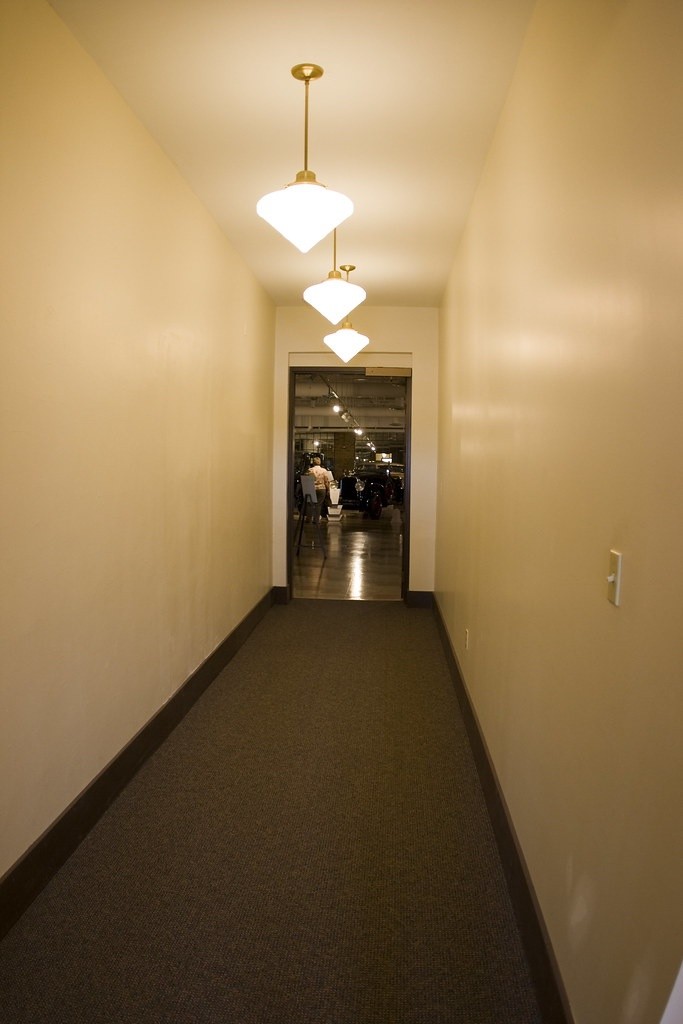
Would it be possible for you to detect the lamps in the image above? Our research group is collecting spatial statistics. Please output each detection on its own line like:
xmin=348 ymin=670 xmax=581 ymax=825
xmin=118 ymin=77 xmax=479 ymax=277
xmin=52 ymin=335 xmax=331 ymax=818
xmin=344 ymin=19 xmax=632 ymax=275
xmin=256 ymin=64 xmax=354 ymax=252
xmin=323 ymin=265 xmax=369 ymax=361
xmin=303 ymin=229 xmax=367 ymax=326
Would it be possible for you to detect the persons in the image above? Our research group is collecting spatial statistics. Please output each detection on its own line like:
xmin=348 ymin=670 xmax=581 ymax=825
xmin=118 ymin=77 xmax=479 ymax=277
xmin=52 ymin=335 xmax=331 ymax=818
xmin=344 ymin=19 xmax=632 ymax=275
xmin=304 ymin=457 xmax=331 ymax=524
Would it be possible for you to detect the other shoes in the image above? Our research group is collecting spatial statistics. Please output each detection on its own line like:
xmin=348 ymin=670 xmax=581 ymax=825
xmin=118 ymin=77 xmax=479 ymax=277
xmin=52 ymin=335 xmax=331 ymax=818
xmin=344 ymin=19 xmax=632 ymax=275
xmin=305 ymin=520 xmax=310 ymax=523
xmin=312 ymin=521 xmax=321 ymax=524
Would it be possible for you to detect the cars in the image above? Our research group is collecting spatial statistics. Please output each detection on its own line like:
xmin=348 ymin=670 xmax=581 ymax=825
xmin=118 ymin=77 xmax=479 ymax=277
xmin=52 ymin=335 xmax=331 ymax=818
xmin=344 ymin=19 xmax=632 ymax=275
xmin=295 ymin=451 xmax=407 ymax=520
